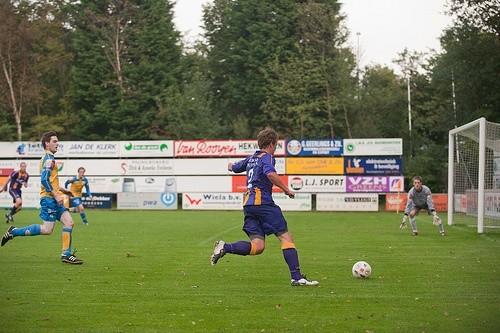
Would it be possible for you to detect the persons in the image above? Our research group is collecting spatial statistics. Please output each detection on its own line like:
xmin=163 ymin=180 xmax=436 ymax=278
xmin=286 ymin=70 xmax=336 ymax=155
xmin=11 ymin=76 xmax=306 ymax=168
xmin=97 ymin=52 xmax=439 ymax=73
xmin=65 ymin=167 xmax=92 ymax=226
xmin=209 ymin=127 xmax=320 ymax=287
xmin=1 ymin=131 xmax=85 ymax=265
xmin=400 ymin=175 xmax=445 ymax=236
xmin=0 ymin=162 xmax=30 ymax=224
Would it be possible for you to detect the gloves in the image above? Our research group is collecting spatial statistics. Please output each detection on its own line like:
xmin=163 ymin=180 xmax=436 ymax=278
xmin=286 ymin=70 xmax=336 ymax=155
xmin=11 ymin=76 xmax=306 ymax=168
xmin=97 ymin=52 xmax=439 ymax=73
xmin=433 ymin=215 xmax=442 ymax=226
xmin=400 ymin=219 xmax=408 ymax=230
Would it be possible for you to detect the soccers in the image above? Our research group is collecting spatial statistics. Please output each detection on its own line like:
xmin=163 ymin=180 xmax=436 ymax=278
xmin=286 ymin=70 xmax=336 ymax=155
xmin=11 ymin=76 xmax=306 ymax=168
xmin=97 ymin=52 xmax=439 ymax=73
xmin=352 ymin=261 xmax=372 ymax=279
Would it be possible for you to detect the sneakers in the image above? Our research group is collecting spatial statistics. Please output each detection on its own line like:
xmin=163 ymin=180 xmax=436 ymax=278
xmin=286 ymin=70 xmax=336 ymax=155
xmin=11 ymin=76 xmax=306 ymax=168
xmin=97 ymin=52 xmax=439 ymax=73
xmin=0 ymin=226 xmax=15 ymax=246
xmin=210 ymin=241 xmax=226 ymax=266
xmin=291 ymin=278 xmax=319 ymax=285
xmin=61 ymin=254 xmax=84 ymax=264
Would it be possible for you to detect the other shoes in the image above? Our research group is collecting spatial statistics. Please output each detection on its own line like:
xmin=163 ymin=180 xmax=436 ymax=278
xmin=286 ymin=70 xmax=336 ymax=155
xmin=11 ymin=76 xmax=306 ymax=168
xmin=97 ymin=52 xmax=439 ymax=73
xmin=5 ymin=213 xmax=14 ymax=223
xmin=440 ymin=230 xmax=446 ymax=235
xmin=411 ymin=231 xmax=417 ymax=236
